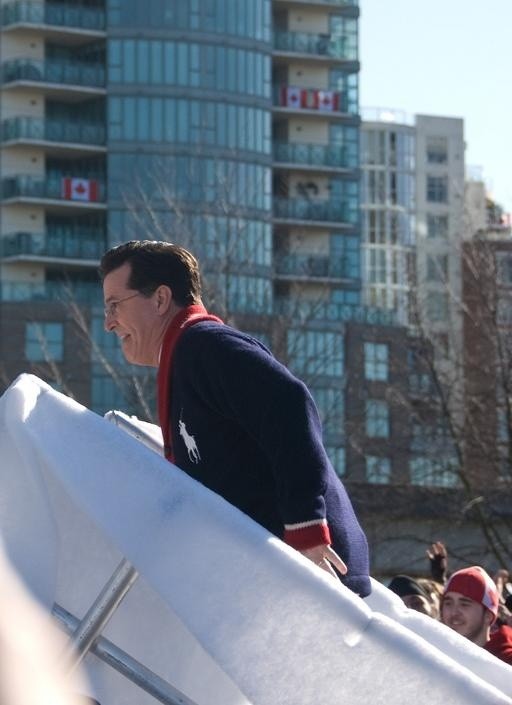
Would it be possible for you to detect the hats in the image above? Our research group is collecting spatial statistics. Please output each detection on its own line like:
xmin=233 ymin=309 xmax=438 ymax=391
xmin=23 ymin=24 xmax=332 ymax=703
xmin=386 ymin=573 xmax=433 ymax=602
xmin=442 ymin=564 xmax=499 ymax=624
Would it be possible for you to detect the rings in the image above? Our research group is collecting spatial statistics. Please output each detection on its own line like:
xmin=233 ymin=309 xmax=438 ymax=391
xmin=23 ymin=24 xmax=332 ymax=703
xmin=318 ymin=560 xmax=324 ymax=566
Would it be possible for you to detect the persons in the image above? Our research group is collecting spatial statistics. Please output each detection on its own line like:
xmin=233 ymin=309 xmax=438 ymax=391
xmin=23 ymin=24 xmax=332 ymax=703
xmin=98 ymin=240 xmax=372 ymax=598
xmin=388 ymin=541 xmax=512 ymax=665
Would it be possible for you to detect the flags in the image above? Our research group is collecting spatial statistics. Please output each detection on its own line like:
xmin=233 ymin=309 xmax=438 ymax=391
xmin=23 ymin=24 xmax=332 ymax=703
xmin=313 ymin=90 xmax=339 ymax=111
xmin=278 ymin=86 xmax=306 ymax=108
xmin=61 ymin=177 xmax=97 ymax=201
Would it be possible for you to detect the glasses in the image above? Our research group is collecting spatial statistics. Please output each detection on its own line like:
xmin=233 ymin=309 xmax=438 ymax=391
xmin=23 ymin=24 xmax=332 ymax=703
xmin=102 ymin=289 xmax=142 ymax=320
